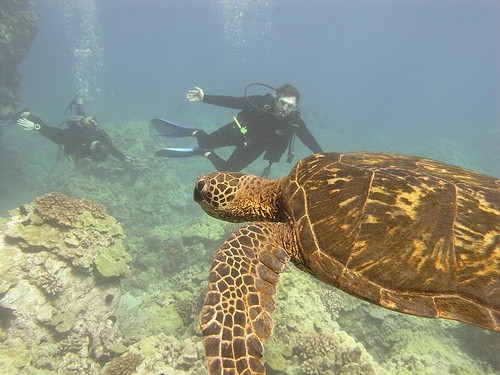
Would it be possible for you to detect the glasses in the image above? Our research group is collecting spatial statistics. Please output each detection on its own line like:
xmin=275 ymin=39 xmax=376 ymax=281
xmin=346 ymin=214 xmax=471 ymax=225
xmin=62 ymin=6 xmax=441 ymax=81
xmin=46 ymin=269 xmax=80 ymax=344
xmin=276 ymin=98 xmax=299 ymax=112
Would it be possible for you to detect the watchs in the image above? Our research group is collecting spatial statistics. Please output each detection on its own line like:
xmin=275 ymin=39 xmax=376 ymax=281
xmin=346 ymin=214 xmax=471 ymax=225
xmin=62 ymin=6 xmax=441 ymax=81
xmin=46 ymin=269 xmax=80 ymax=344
xmin=34 ymin=124 xmax=41 ymax=131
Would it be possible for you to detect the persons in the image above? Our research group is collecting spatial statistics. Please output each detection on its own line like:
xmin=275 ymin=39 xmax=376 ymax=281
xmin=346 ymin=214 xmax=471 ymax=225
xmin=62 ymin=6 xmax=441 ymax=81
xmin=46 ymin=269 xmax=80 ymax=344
xmin=151 ymin=83 xmax=323 ymax=172
xmin=12 ymin=96 xmax=135 ymax=164
